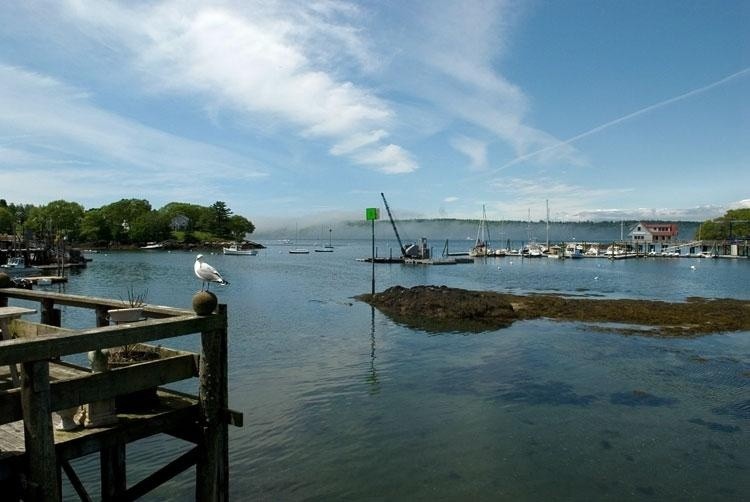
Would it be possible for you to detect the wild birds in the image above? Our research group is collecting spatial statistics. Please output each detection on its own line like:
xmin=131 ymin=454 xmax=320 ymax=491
xmin=194 ymin=254 xmax=231 ymax=293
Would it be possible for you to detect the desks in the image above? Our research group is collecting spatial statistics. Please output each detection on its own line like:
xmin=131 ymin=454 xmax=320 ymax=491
xmin=0 ymin=305 xmax=38 ymax=389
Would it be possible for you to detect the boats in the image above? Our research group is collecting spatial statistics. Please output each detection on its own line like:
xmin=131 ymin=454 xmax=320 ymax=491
xmin=289 ymin=248 xmax=309 ymax=254
xmin=314 ymin=245 xmax=334 ymax=252
xmin=140 ymin=242 xmax=164 ymax=250
xmin=223 ymin=245 xmax=258 ymax=256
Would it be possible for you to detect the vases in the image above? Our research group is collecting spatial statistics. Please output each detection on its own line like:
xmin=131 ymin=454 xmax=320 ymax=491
xmin=88 ymin=351 xmax=162 ymax=412
xmin=109 ymin=306 xmax=142 ymax=324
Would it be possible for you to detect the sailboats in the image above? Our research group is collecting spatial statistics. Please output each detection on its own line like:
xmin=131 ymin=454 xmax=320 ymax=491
xmin=470 ymin=199 xmax=644 ymax=259
